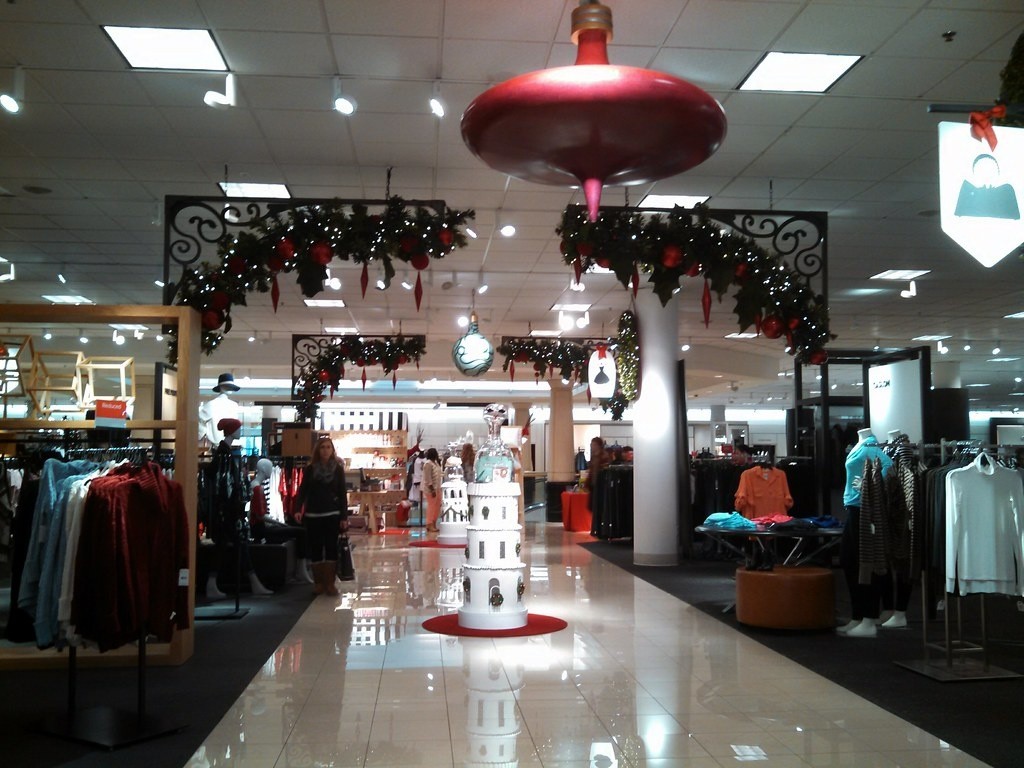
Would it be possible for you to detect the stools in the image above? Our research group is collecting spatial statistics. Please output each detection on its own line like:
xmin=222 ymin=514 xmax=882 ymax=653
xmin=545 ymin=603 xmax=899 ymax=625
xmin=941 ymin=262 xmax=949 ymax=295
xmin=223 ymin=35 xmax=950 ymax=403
xmin=735 ymin=567 xmax=833 ymax=630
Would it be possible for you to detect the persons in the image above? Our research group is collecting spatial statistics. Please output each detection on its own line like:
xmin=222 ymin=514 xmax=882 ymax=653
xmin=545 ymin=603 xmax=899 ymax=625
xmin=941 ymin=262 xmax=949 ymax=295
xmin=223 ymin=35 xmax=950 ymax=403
xmin=198 ymin=371 xmax=315 ymax=598
xmin=461 ymin=443 xmax=475 ymax=507
xmin=732 ymin=444 xmax=752 ymax=467
xmin=405 ymin=452 xmax=425 ymax=496
xmin=586 ymin=437 xmax=633 ymax=536
xmin=836 ymin=427 xmax=914 ymax=636
xmin=294 ymin=437 xmax=349 ymax=595
xmin=425 ymin=448 xmax=443 ymax=531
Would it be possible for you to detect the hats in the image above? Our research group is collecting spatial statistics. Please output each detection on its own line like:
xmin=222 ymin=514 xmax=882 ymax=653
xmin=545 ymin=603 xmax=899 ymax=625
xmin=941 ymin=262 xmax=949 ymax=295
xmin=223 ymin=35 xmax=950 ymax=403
xmin=212 ymin=373 xmax=240 ymax=392
xmin=218 ymin=419 xmax=242 ymax=436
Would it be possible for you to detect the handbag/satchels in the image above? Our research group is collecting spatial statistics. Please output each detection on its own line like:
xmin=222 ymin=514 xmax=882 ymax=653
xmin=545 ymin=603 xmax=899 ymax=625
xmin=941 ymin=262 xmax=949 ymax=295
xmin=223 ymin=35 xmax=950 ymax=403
xmin=335 ymin=609 xmax=354 ymax=665
xmin=475 ymin=436 xmax=514 ymax=482
xmin=338 ymin=532 xmax=354 ymax=581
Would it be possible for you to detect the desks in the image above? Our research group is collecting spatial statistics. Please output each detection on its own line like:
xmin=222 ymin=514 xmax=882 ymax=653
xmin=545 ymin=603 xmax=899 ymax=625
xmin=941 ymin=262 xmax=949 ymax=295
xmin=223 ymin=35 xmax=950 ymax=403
xmin=561 ymin=491 xmax=593 ymax=532
xmin=246 ymin=538 xmax=297 ymax=582
xmin=347 ymin=489 xmax=387 ymax=533
xmin=695 ymin=525 xmax=843 ymax=614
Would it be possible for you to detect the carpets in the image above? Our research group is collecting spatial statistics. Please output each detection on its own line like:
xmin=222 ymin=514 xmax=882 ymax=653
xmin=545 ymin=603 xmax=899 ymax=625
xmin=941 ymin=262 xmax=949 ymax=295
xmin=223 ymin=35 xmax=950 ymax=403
xmin=0 ymin=542 xmax=357 ymax=768
xmin=577 ymin=538 xmax=1024 ymax=768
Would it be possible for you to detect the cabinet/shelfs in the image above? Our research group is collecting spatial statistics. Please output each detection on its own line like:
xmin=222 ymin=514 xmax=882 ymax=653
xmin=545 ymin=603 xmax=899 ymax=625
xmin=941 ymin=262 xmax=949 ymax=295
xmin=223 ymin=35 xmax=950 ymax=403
xmin=318 ymin=429 xmax=408 ymax=534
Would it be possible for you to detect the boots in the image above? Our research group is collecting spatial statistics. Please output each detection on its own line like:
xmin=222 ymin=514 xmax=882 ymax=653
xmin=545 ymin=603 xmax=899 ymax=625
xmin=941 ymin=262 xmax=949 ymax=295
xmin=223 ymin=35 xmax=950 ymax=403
xmin=325 ymin=561 xmax=337 ymax=596
xmin=311 ymin=561 xmax=324 ymax=594
xmin=426 ymin=521 xmax=439 ymax=532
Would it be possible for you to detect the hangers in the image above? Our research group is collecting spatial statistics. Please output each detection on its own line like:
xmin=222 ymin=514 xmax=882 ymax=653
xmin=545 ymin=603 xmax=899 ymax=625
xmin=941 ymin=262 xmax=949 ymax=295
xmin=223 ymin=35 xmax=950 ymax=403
xmin=0 ymin=437 xmax=1024 ymax=474
